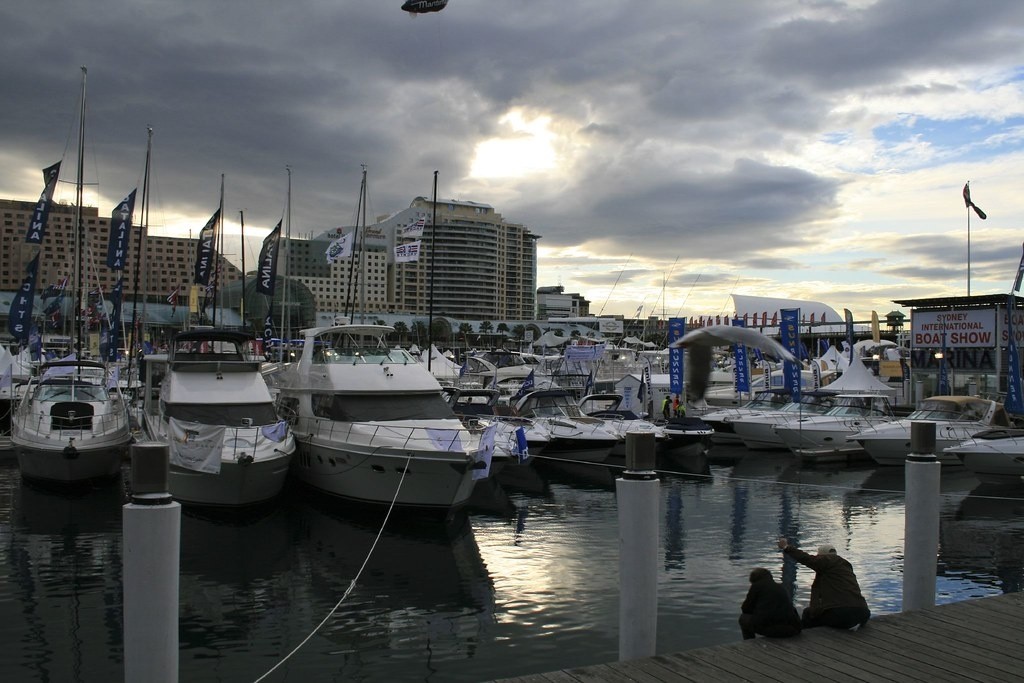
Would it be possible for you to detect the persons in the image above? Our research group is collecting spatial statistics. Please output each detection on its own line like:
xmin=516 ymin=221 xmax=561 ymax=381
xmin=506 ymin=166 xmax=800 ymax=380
xmin=778 ymin=538 xmax=871 ymax=632
xmin=739 ymin=568 xmax=802 ymax=640
xmin=661 ymin=395 xmax=687 ymax=419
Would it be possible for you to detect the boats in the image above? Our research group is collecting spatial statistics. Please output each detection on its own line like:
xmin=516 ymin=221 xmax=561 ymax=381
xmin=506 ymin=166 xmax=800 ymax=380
xmin=943 ymin=427 xmax=1024 ymax=476
xmin=847 ymin=394 xmax=1018 ymax=467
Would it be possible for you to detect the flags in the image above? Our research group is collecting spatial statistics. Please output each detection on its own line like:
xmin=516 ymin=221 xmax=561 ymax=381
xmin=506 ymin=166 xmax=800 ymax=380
xmin=962 ymin=184 xmax=986 ymax=220
xmin=168 ymin=417 xmax=226 ymax=474
xmin=394 ymin=240 xmax=422 ymax=262
xmin=42 ymin=274 xmax=178 ymax=332
xmin=425 ymin=427 xmax=464 ymax=451
xmin=402 ymin=217 xmax=426 ymax=238
xmin=472 ymin=424 xmax=497 ymax=480
xmin=325 ymin=232 xmax=353 ymax=262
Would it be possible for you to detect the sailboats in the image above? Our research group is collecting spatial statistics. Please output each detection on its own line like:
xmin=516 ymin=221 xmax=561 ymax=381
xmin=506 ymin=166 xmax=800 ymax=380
xmin=0 ymin=65 xmax=910 ymax=521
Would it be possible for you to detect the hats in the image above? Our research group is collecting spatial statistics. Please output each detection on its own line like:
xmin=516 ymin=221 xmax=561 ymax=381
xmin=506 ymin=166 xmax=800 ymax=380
xmin=817 ymin=545 xmax=837 ymax=556
xmin=750 ymin=568 xmax=772 ymax=583
